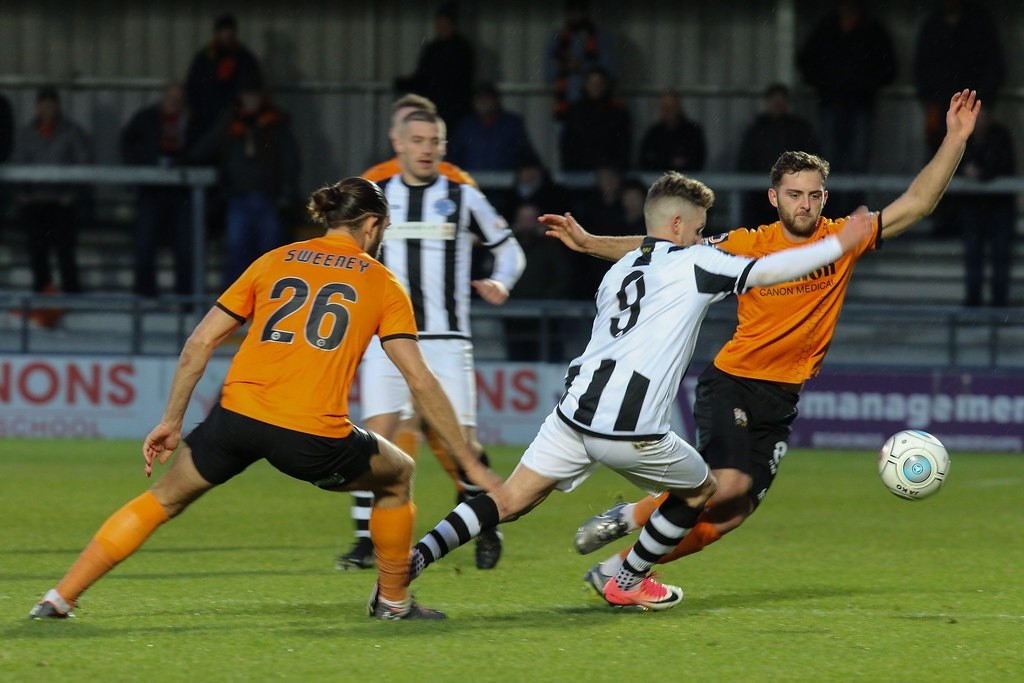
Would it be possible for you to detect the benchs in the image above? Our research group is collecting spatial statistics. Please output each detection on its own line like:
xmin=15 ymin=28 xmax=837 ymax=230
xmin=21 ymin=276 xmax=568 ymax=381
xmin=3 ymin=196 xmax=1022 ymax=371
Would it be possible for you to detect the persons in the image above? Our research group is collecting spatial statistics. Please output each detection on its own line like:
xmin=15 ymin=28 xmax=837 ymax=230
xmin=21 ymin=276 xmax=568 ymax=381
xmin=369 ymin=171 xmax=875 ymax=617
xmin=29 ymin=174 xmax=507 ymax=621
xmin=335 ymin=109 xmax=528 ymax=571
xmin=351 ymin=93 xmax=479 ymax=512
xmin=1 ymin=0 xmax=1023 ymax=367
xmin=536 ymin=87 xmax=982 ymax=603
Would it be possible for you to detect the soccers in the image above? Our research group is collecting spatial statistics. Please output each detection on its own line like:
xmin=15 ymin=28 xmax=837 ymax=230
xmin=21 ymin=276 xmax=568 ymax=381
xmin=877 ymin=430 xmax=951 ymax=500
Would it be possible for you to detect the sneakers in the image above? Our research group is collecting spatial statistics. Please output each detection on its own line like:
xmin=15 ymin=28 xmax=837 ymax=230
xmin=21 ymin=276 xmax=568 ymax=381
xmin=28 ymin=600 xmax=70 ymax=620
xmin=601 ymin=575 xmax=686 ymax=610
xmin=575 ymin=501 xmax=633 ymax=553
xmin=584 ymin=564 xmax=644 ymax=609
xmin=370 ymin=595 xmax=451 ymax=622
xmin=476 ymin=529 xmax=501 ymax=571
xmin=334 ymin=537 xmax=378 ymax=570
